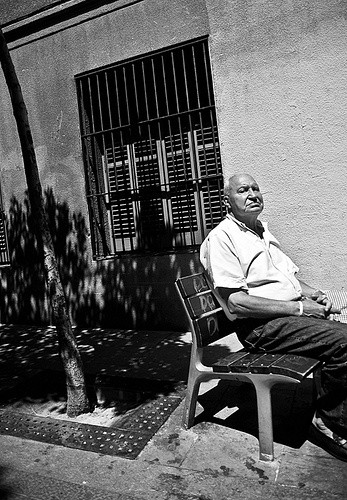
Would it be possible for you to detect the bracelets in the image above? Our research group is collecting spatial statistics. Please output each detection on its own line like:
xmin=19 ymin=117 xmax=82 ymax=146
xmin=313 ymin=290 xmax=320 ymax=295
xmin=297 ymin=301 xmax=303 ymax=316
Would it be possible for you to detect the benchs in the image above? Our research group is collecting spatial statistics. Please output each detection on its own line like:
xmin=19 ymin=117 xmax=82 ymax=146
xmin=175 ymin=272 xmax=321 ymax=462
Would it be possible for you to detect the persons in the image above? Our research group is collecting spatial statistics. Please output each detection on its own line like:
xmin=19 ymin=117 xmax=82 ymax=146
xmin=199 ymin=174 xmax=347 ymax=461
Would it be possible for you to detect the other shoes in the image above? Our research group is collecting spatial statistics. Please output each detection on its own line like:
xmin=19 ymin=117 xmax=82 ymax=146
xmin=308 ymin=419 xmax=347 ymax=462
